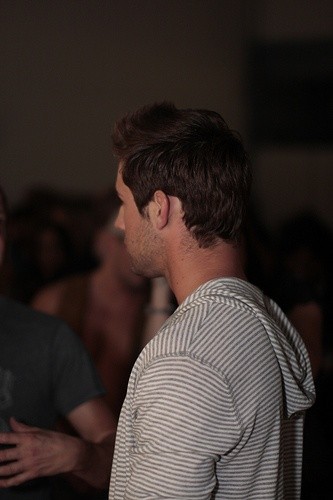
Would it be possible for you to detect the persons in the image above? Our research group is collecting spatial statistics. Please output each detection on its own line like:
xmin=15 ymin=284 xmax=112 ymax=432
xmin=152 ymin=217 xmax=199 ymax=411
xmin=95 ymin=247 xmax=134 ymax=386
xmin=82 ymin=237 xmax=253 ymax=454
xmin=0 ymin=97 xmax=333 ymax=500
